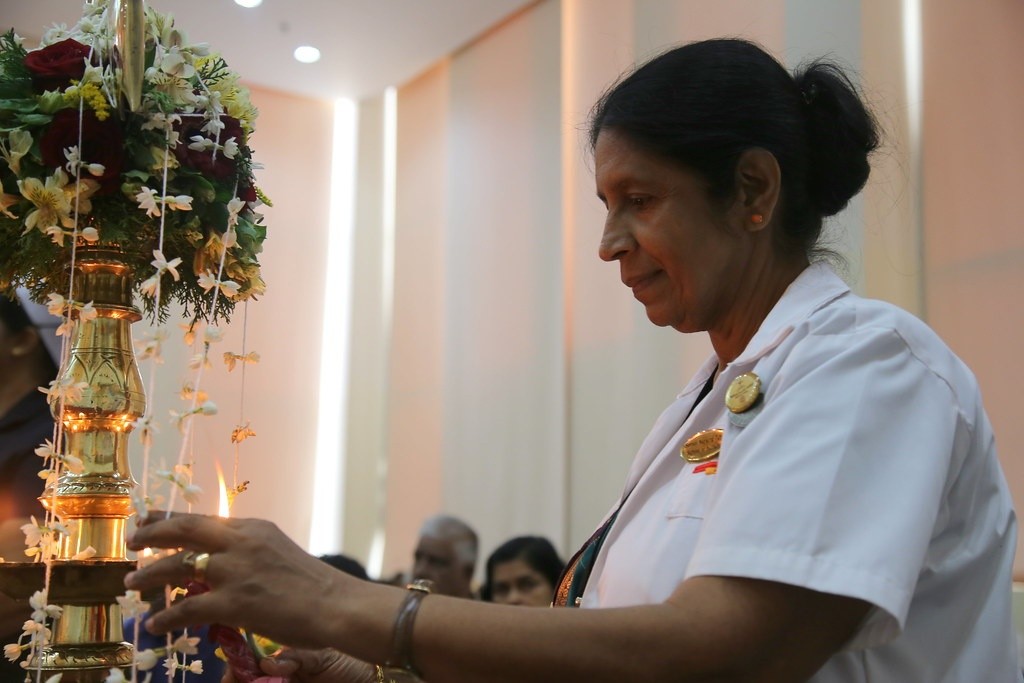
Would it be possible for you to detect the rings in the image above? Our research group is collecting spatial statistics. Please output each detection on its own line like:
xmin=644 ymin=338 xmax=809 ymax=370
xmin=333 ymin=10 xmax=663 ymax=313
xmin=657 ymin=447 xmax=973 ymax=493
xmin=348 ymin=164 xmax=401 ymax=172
xmin=194 ymin=552 xmax=211 ymax=582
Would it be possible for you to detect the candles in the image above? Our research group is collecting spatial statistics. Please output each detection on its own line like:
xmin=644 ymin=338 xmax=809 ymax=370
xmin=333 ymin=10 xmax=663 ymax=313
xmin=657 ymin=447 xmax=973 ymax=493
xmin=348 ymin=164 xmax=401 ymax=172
xmin=186 ymin=581 xmax=259 ymax=683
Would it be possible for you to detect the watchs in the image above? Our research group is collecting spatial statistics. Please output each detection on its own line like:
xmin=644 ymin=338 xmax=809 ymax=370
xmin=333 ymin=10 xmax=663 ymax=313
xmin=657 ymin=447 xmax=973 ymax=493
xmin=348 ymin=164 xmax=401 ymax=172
xmin=380 ymin=580 xmax=434 ymax=667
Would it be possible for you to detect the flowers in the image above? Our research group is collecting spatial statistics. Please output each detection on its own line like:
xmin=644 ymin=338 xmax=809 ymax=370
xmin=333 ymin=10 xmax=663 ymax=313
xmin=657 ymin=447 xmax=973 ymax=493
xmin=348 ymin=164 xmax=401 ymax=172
xmin=0 ymin=0 xmax=274 ymax=683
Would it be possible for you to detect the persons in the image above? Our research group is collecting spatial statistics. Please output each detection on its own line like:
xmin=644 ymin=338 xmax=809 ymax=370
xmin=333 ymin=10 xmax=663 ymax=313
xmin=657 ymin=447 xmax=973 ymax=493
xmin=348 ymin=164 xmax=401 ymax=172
xmin=0 ymin=284 xmax=77 ymax=523
xmin=117 ymin=518 xmax=567 ymax=683
xmin=128 ymin=37 xmax=1016 ymax=682
